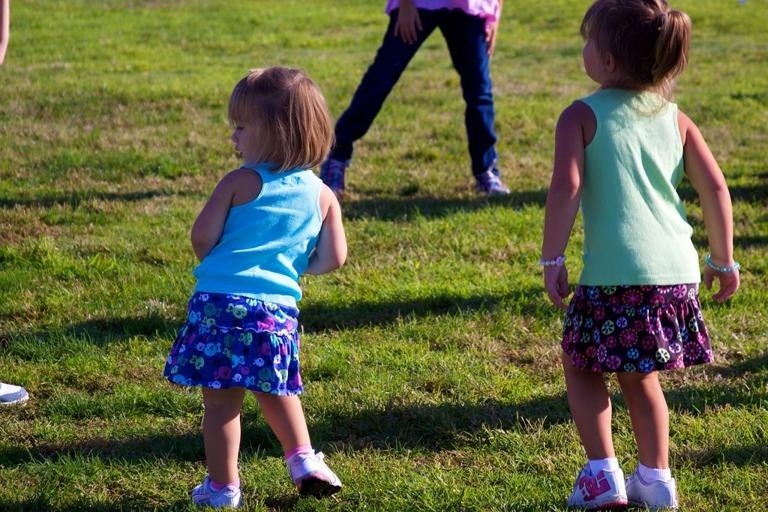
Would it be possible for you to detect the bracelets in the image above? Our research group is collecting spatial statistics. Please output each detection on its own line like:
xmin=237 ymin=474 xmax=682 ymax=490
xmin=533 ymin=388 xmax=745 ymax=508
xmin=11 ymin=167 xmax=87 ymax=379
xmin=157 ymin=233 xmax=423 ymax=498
xmin=703 ymin=254 xmax=740 ymax=273
xmin=539 ymin=257 xmax=570 ymax=266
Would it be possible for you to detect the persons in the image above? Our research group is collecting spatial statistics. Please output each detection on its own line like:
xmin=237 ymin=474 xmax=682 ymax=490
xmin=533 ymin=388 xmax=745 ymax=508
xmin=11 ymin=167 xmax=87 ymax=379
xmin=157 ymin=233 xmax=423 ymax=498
xmin=319 ymin=0 xmax=517 ymax=201
xmin=540 ymin=1 xmax=741 ymax=510
xmin=0 ymin=0 xmax=31 ymax=405
xmin=164 ymin=66 xmax=348 ymax=509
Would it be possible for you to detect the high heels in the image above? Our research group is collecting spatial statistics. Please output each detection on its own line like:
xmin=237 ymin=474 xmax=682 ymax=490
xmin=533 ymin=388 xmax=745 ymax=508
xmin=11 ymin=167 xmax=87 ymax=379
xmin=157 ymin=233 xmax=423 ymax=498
xmin=0 ymin=382 xmax=29 ymax=406
xmin=319 ymin=159 xmax=345 ymax=190
xmin=192 ymin=475 xmax=241 ymax=509
xmin=288 ymin=451 xmax=342 ymax=496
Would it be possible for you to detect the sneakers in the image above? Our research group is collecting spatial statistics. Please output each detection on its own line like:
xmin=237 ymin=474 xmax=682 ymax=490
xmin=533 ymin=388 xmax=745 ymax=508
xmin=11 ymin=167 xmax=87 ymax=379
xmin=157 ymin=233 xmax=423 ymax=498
xmin=477 ymin=170 xmax=510 ymax=195
xmin=568 ymin=461 xmax=677 ymax=510
